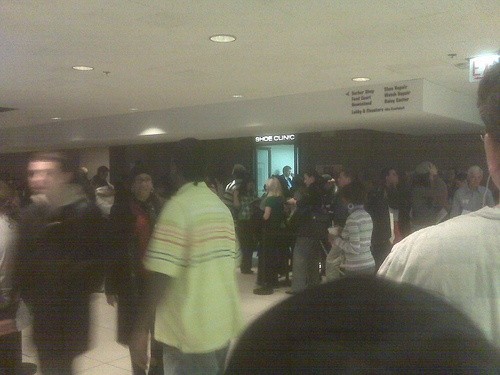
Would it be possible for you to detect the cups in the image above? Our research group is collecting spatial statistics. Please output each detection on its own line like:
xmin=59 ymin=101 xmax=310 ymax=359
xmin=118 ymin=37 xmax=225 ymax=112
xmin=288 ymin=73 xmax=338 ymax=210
xmin=327 ymin=227 xmax=339 ymax=236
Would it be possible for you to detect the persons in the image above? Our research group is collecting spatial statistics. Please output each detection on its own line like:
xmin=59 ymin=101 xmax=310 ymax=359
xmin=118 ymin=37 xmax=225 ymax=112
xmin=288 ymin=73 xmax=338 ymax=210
xmin=379 ymin=62 xmax=500 ymax=347
xmin=104 ymin=165 xmax=164 ymax=375
xmin=18 ymin=152 xmax=105 ymax=375
xmin=223 ymin=273 xmax=500 ymax=374
xmin=254 ymin=165 xmax=375 ymax=298
xmin=93 ymin=167 xmax=165 ymax=216
xmin=0 ymin=183 xmax=25 ymax=375
xmin=377 ymin=161 xmax=494 ymax=257
xmin=224 ymin=165 xmax=255 ymax=274
xmin=328 ymin=183 xmax=376 ymax=277
xmin=129 ymin=139 xmax=238 ymax=375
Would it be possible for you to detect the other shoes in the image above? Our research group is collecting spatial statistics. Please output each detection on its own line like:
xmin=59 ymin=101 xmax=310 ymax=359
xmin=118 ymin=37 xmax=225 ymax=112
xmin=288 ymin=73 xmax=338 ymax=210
xmin=253 ymin=285 xmax=273 ymax=295
xmin=241 ymin=267 xmax=253 ymax=273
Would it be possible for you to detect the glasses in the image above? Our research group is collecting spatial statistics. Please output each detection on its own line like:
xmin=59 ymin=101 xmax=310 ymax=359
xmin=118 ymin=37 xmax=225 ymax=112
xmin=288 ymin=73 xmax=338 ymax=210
xmin=480 ymin=126 xmax=496 ymax=142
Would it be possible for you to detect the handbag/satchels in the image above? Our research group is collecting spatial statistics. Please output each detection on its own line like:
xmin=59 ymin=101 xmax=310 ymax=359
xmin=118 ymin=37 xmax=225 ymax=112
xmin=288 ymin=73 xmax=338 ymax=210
xmin=324 ymin=243 xmax=340 ymax=289
